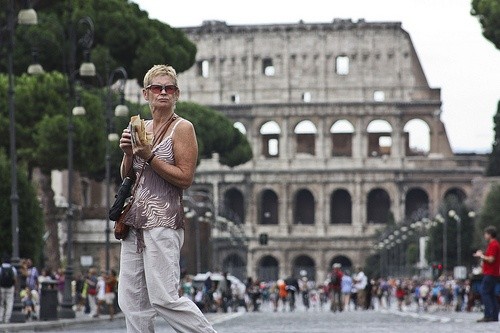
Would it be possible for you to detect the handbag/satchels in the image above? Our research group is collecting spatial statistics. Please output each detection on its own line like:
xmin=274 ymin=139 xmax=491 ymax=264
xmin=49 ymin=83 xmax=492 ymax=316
xmin=108 ymin=174 xmax=132 ymax=220
xmin=114 ymin=196 xmax=134 ymax=240
xmin=406 ymin=299 xmax=411 ymax=305
xmin=82 ymin=282 xmax=87 ymax=298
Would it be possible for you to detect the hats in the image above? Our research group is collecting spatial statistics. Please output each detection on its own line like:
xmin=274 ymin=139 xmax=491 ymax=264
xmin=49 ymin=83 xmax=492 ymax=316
xmin=10 ymin=257 xmax=21 ymax=266
xmin=300 ymin=270 xmax=307 ymax=277
xmin=334 ymin=263 xmax=341 ymax=268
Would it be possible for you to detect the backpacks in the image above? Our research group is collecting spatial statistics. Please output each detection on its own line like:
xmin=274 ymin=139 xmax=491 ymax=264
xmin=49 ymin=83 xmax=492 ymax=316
xmin=301 ymin=279 xmax=309 ymax=291
xmin=332 ymin=272 xmax=338 ymax=284
xmin=0 ymin=266 xmax=16 ymax=288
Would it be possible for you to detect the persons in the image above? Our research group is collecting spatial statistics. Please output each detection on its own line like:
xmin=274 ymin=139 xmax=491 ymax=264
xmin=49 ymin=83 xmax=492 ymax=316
xmin=0 ymin=257 xmax=123 ymax=325
xmin=179 ymin=225 xmax=500 ymax=322
xmin=117 ymin=65 xmax=217 ymax=333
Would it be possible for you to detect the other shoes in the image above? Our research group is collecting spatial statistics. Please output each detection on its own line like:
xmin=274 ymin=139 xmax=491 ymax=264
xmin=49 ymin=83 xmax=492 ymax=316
xmin=476 ymin=315 xmax=498 ymax=322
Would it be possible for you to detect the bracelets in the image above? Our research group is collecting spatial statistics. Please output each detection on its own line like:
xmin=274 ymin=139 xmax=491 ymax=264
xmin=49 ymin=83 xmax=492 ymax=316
xmin=147 ymin=153 xmax=156 ymax=164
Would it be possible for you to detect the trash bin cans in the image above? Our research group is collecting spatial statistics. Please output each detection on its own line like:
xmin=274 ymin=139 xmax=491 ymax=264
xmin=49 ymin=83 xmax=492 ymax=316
xmin=40 ymin=280 xmax=58 ymax=319
xmin=113 ymin=289 xmax=121 ymax=312
xmin=471 ymin=274 xmax=483 ymax=307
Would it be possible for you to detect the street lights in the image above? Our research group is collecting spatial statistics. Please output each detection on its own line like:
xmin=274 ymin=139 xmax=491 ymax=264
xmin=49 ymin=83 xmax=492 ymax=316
xmin=180 ymin=191 xmax=249 ymax=309
xmin=373 ymin=202 xmax=475 ymax=316
xmin=100 ymin=49 xmax=129 ymax=314
xmin=0 ymin=0 xmax=39 ymax=323
xmin=58 ymin=0 xmax=100 ymax=319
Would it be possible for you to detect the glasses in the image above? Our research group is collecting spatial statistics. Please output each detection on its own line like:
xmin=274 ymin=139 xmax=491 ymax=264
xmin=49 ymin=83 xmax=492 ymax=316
xmin=146 ymin=84 xmax=179 ymax=94
